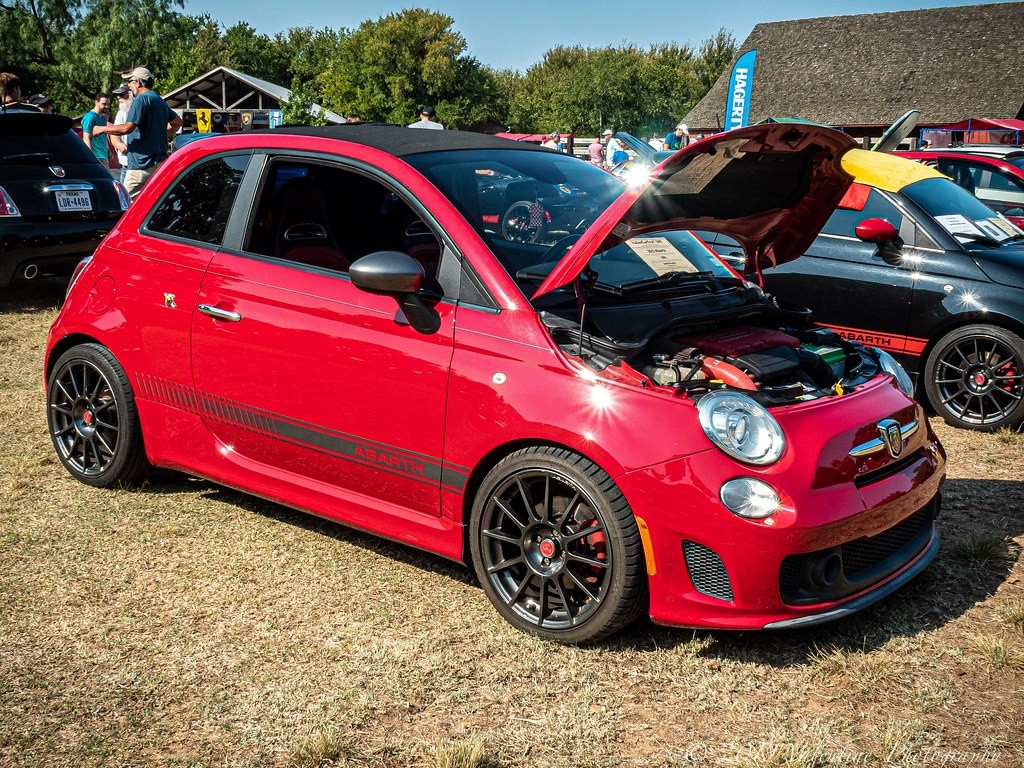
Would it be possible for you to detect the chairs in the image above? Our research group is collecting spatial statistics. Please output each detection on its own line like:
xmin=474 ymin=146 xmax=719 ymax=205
xmin=271 ymin=176 xmax=352 ymax=273
xmin=392 ymin=196 xmax=461 ymax=299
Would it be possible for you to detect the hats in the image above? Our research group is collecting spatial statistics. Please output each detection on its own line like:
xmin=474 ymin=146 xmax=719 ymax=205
xmin=28 ymin=94 xmax=53 ymax=106
xmin=422 ymin=106 xmax=436 ymax=117
xmin=602 ymin=129 xmax=612 ymax=135
xmin=677 ymin=124 xmax=690 ymax=135
xmin=121 ymin=67 xmax=154 ymax=81
xmin=111 ymin=81 xmax=130 ymax=93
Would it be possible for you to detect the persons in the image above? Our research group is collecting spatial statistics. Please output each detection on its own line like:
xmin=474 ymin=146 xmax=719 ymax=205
xmin=29 ymin=94 xmax=54 ymax=114
xmin=610 ymin=142 xmax=629 ymax=166
xmin=93 ymin=67 xmax=182 ymax=201
xmin=81 ymin=93 xmax=110 ymax=170
xmin=0 ymin=73 xmax=42 ymax=112
xmin=588 ymin=138 xmax=604 ymax=164
xmin=103 ymin=111 xmax=122 ymax=168
xmin=110 ymin=82 xmax=134 ymax=183
xmin=662 ymin=124 xmax=689 ymax=150
xmin=648 ymin=132 xmax=663 ymax=151
xmin=346 ymin=114 xmax=362 ymax=123
xmin=918 ymin=140 xmax=928 ymax=165
xmin=602 ymin=130 xmax=620 ymax=170
xmin=695 ymin=134 xmax=705 ymax=140
xmin=408 ymin=107 xmax=444 ymax=130
xmin=540 ymin=133 xmax=564 ymax=152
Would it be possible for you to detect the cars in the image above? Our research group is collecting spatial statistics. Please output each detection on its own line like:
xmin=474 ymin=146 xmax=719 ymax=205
xmin=43 ymin=122 xmax=949 ymax=646
xmin=575 ymin=149 xmax=1024 ymax=434
xmin=886 ymin=146 xmax=1024 ymax=232
xmin=476 ymin=161 xmax=601 ymax=245
xmin=0 ymin=108 xmax=132 ymax=290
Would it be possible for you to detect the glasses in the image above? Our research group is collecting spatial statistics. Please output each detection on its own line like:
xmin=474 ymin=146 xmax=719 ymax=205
xmin=38 ymin=102 xmax=50 ymax=108
xmin=114 ymin=92 xmax=127 ymax=98
